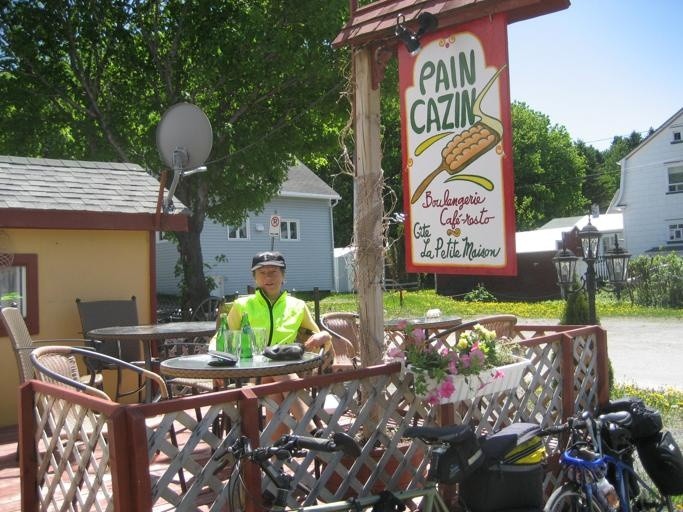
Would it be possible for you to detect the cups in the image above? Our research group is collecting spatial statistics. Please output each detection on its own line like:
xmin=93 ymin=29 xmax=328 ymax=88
xmin=223 ymin=326 xmax=269 ymax=363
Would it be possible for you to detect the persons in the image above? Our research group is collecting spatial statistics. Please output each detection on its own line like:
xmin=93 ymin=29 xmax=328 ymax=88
xmin=209 ymin=251 xmax=335 ymax=476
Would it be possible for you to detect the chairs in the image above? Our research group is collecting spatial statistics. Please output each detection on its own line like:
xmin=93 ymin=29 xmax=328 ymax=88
xmin=2 ymin=296 xmax=512 ymax=500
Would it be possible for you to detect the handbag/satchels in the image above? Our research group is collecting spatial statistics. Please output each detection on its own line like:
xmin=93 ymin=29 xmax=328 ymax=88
xmin=262 ymin=344 xmax=304 ymax=359
xmin=595 ymin=398 xmax=683 ymax=500
xmin=460 ymin=422 xmax=543 ymax=512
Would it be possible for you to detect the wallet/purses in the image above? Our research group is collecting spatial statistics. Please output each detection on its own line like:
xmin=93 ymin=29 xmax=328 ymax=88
xmin=208 ymin=351 xmax=238 ymax=366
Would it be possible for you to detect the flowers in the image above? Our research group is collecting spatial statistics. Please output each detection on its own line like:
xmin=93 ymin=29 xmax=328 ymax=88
xmin=386 ymin=313 xmax=527 ymax=408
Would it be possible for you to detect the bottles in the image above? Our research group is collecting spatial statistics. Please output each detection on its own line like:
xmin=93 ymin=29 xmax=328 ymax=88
xmin=214 ymin=312 xmax=255 ymax=360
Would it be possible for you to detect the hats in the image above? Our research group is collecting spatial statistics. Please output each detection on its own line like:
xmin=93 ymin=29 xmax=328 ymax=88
xmin=252 ymin=253 xmax=286 ymax=271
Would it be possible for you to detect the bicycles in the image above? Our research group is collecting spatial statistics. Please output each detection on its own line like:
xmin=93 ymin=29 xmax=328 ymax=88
xmin=536 ymin=396 xmax=683 ymax=512
xmin=213 ymin=418 xmax=549 ymax=511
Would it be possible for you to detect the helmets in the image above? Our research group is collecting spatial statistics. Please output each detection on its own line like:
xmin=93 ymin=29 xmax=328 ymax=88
xmin=559 ymin=447 xmax=608 ymax=487
